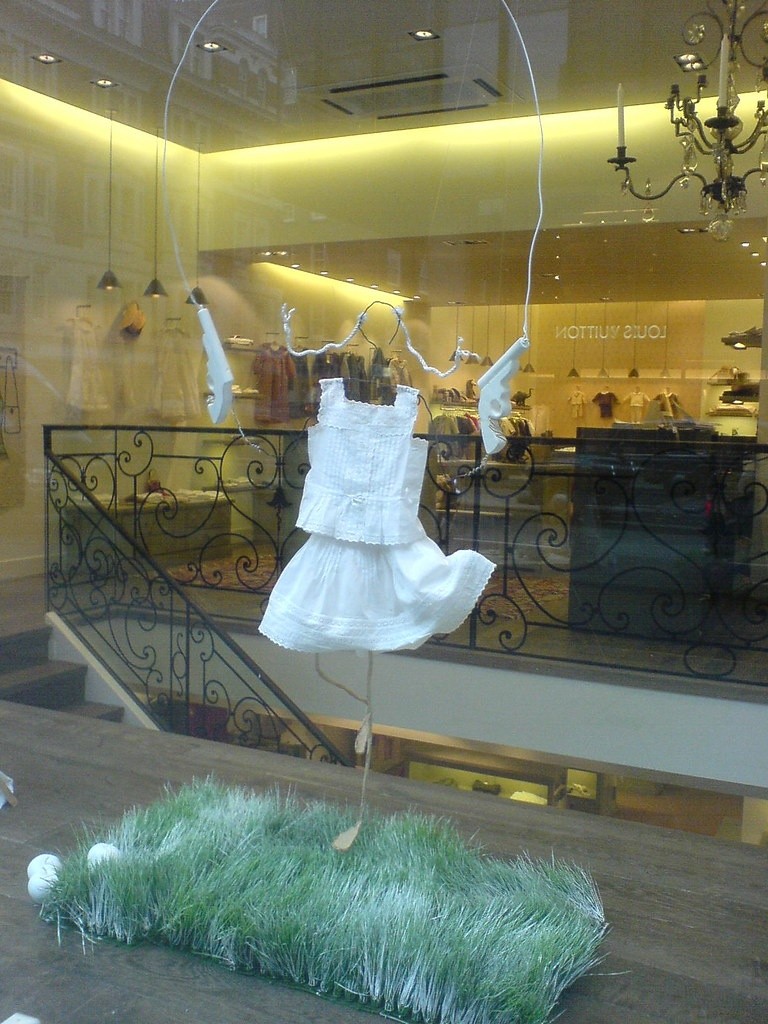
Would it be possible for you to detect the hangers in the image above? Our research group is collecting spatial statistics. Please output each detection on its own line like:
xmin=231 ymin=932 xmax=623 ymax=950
xmin=66 ymin=305 xmax=102 ymax=328
xmin=269 ymin=335 xmax=404 ymax=365
xmin=441 ymin=409 xmax=527 ymax=422
xmin=164 ymin=316 xmax=192 ymax=339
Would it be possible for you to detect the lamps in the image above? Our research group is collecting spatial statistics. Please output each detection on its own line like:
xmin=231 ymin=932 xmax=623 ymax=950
xmin=94 ymin=109 xmax=123 ymax=291
xmin=628 ymin=301 xmax=640 ymax=378
xmin=566 ymin=304 xmax=581 ymax=378
xmin=448 ymin=305 xmax=464 ymax=361
xmin=659 ymin=301 xmax=672 ymax=378
xmin=479 ymin=305 xmax=495 ymax=367
xmin=606 ymin=0 xmax=768 ymax=242
xmin=142 ymin=125 xmax=171 ymax=298
xmin=522 ymin=306 xmax=535 ymax=373
xmin=597 ymin=303 xmax=610 ymax=378
xmin=185 ymin=139 xmax=209 ymax=306
xmin=464 ymin=306 xmax=480 ymax=365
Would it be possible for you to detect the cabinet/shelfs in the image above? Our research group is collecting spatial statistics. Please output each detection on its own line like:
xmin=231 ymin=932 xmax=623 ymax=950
xmin=65 ymin=497 xmax=237 ymax=576
xmin=435 ymin=459 xmax=544 ymax=573
xmin=706 ymin=381 xmax=758 ymax=418
xmin=718 ymin=342 xmax=762 ymax=402
xmin=201 ymin=346 xmax=269 ymax=494
